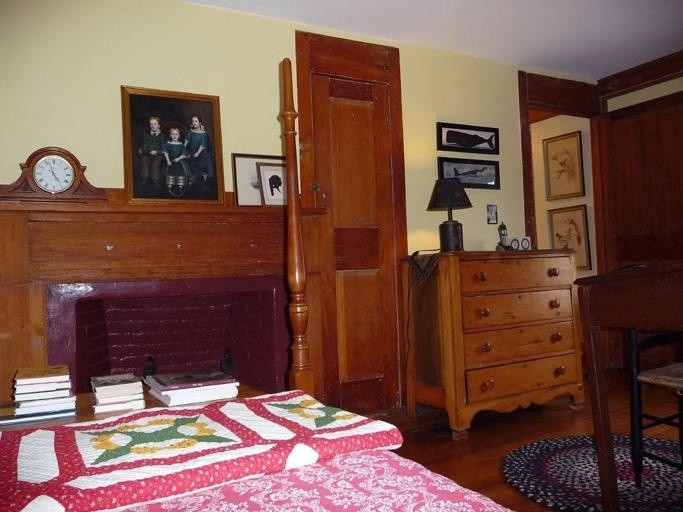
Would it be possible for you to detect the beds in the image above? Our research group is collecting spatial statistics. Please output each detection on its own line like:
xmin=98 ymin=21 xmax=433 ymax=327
xmin=0 ymin=56 xmax=518 ymax=512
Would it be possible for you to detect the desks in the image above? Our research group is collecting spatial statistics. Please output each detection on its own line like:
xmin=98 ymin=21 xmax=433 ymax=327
xmin=576 ymin=259 xmax=682 ymax=511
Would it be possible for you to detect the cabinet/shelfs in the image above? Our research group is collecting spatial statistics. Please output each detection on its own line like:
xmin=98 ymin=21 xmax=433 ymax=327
xmin=404 ymin=247 xmax=586 ymax=433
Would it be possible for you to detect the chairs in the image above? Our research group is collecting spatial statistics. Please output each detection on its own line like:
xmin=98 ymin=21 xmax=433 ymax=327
xmin=626 ymin=325 xmax=681 ymax=487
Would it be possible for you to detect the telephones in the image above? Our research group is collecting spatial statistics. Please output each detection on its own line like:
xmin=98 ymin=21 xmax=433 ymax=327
xmin=496 ymin=222 xmax=516 ymax=252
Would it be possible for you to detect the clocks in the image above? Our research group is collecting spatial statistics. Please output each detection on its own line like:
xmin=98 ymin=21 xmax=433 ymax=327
xmin=2 ymin=142 xmax=106 ymax=199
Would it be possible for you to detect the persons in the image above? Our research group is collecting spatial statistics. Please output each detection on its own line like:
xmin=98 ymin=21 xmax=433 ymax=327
xmin=182 ymin=115 xmax=213 ymax=185
xmin=163 ymin=128 xmax=191 ymax=192
xmin=138 ymin=117 xmax=167 ymax=187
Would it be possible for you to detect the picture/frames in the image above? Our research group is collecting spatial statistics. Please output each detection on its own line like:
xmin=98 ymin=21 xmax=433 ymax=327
xmin=229 ymin=153 xmax=287 ymax=207
xmin=546 ymin=204 xmax=592 ymax=271
xmin=435 ymin=121 xmax=500 ymax=155
xmin=436 ymin=157 xmax=501 ymax=190
xmin=254 ymin=160 xmax=288 ymax=208
xmin=121 ymin=82 xmax=227 ymax=208
xmin=540 ymin=129 xmax=585 ymax=201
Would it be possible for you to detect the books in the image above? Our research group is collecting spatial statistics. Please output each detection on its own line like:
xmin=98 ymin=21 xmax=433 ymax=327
xmin=0 ymin=365 xmax=241 ymax=427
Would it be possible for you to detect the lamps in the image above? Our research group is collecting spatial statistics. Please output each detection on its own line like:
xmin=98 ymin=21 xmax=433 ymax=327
xmin=427 ymin=178 xmax=474 ymax=250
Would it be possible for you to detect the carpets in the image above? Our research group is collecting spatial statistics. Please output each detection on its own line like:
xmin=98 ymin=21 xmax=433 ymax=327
xmin=500 ymin=431 xmax=682 ymax=512
